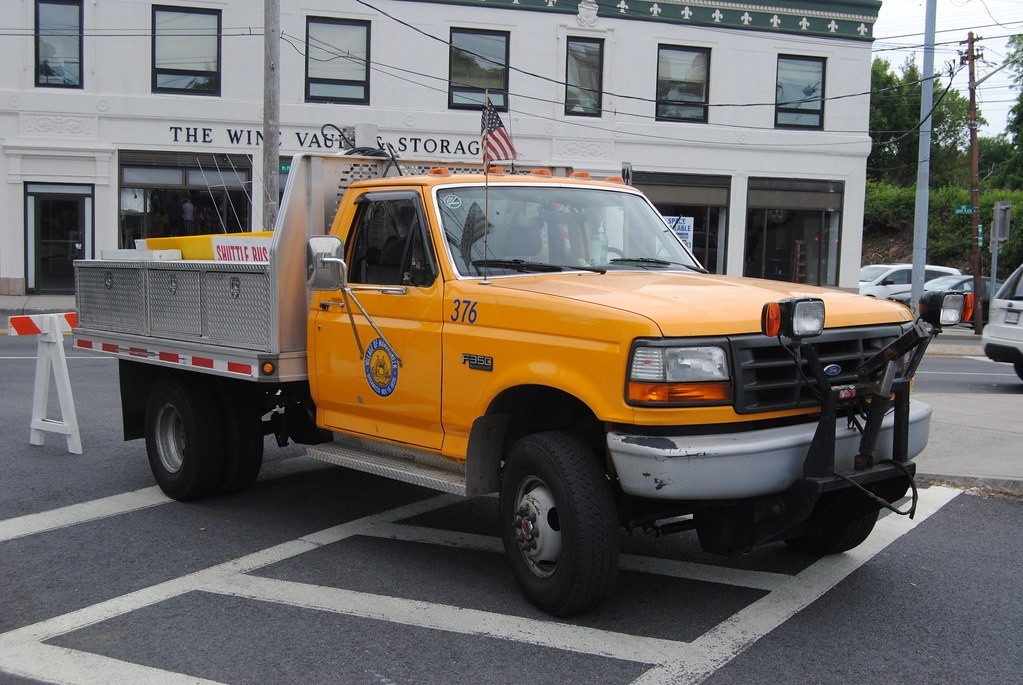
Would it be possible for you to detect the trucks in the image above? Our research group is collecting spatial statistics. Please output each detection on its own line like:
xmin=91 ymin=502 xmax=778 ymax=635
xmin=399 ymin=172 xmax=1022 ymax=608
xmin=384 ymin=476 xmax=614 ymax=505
xmin=71 ymin=164 xmax=940 ymax=618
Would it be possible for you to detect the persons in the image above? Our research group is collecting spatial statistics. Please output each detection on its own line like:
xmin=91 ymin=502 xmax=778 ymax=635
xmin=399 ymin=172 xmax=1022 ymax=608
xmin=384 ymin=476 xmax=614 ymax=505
xmin=379 ymin=207 xmax=427 ymax=269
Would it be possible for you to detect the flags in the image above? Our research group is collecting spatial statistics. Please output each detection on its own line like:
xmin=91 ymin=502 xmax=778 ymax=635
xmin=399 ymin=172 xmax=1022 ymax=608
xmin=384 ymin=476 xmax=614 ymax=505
xmin=480 ymin=97 xmax=522 ymax=175
xmin=459 ymin=201 xmax=494 ymax=277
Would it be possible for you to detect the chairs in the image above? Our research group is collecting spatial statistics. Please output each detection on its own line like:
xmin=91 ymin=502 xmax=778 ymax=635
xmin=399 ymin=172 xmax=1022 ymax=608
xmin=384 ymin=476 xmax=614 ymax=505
xmin=503 ymin=225 xmax=543 ymax=261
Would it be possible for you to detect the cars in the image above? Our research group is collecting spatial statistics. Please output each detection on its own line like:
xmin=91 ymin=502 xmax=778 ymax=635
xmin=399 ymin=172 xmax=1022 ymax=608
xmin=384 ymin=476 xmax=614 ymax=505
xmin=886 ymin=274 xmax=1004 ymax=329
xmin=858 ymin=262 xmax=972 ymax=302
xmin=981 ymin=263 xmax=1023 ymax=381
